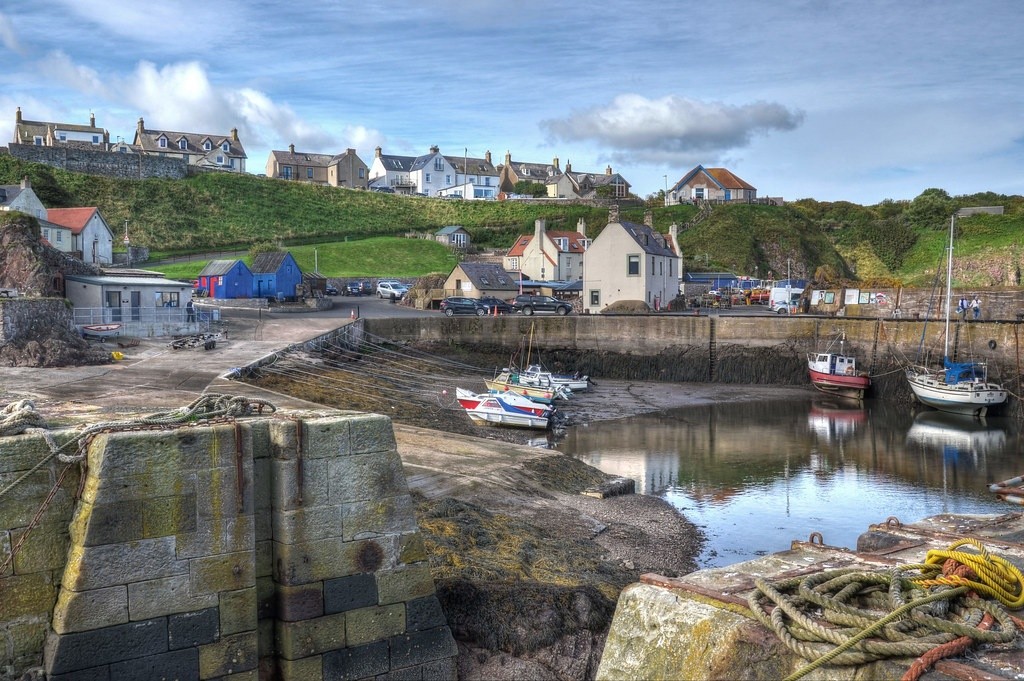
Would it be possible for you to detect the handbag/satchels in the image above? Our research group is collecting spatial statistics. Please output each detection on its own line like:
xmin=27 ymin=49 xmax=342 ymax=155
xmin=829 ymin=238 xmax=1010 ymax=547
xmin=973 ymin=307 xmax=977 ymax=311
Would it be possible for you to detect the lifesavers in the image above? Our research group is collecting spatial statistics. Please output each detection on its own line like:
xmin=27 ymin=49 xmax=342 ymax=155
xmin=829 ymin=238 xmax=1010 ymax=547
xmin=989 ymin=339 xmax=997 ymax=350
xmin=845 ymin=366 xmax=855 ymax=375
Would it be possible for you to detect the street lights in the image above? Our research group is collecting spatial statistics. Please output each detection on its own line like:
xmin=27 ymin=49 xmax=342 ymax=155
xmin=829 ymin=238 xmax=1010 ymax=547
xmin=662 ymin=174 xmax=668 ymax=207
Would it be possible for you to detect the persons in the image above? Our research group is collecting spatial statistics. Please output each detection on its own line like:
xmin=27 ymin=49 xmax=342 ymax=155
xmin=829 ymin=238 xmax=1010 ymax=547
xmin=959 ymin=294 xmax=970 ymax=319
xmin=970 ymin=296 xmax=982 ymax=319
xmin=186 ymin=299 xmax=195 ymax=323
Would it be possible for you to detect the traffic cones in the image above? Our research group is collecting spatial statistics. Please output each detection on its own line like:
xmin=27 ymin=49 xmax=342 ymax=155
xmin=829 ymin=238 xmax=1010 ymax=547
xmin=493 ymin=304 xmax=498 ymax=316
xmin=350 ymin=309 xmax=356 ymax=319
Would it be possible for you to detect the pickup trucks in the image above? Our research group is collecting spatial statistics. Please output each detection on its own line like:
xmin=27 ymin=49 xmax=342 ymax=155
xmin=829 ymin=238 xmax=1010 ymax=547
xmin=510 ymin=293 xmax=573 ymax=316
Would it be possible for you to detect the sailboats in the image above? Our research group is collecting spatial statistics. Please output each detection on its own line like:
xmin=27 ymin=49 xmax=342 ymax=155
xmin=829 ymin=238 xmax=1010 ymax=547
xmin=906 ymin=409 xmax=1008 ymax=500
xmin=902 ymin=214 xmax=1012 ymax=420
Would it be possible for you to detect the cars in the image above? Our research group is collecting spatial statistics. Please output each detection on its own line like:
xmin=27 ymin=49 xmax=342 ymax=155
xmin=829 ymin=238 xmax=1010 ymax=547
xmin=341 ymin=281 xmax=362 ymax=296
xmin=376 ymin=281 xmax=409 ymax=299
xmin=358 ymin=281 xmax=373 ymax=295
xmin=406 ymin=284 xmax=414 ymax=288
xmin=769 ymin=301 xmax=793 ymax=314
xmin=373 ymin=186 xmax=395 ymax=193
xmin=438 ymin=194 xmax=464 ymax=199
xmin=481 ymin=298 xmax=516 ymax=314
xmin=438 ymin=296 xmax=488 ymax=317
xmin=325 ymin=284 xmax=337 ymax=296
xmin=409 ymin=192 xmax=426 ymax=198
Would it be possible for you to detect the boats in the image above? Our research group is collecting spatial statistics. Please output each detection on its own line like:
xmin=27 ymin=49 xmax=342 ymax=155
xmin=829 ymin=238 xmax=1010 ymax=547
xmin=483 ymin=320 xmax=589 ymax=404
xmin=462 ymin=395 xmax=550 ymax=430
xmin=83 ymin=323 xmax=123 ymax=337
xmin=807 ymin=400 xmax=867 ymax=431
xmin=455 ymin=386 xmax=554 ymax=418
xmin=807 ymin=329 xmax=873 ymax=399
